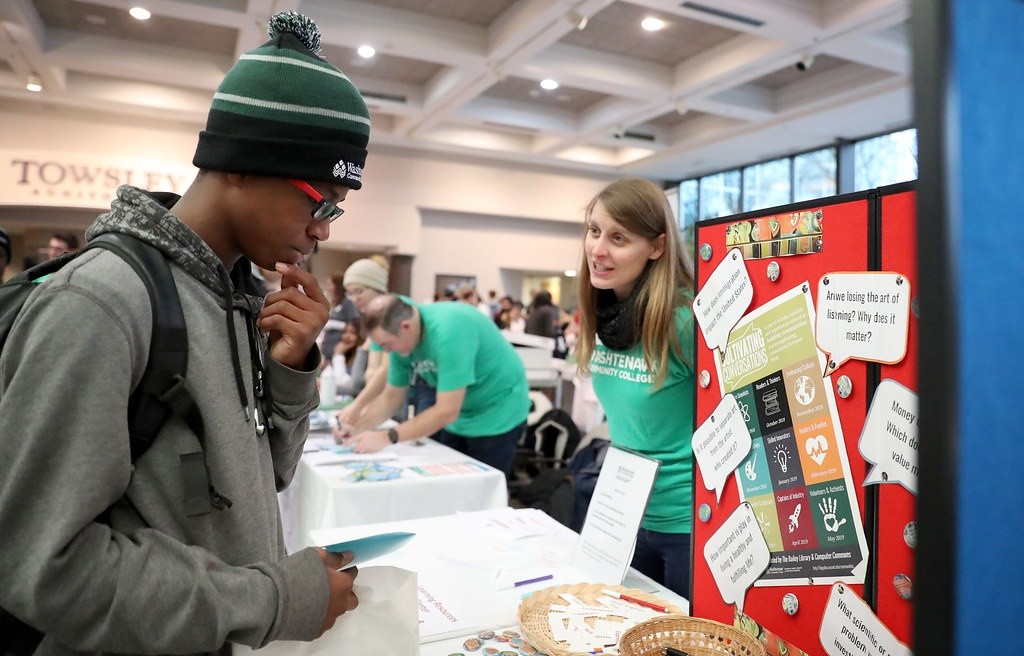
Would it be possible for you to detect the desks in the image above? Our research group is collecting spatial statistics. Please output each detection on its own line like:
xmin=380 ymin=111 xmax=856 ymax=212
xmin=306 ymin=504 xmax=690 ymax=655
xmin=277 ymin=416 xmax=510 ymax=557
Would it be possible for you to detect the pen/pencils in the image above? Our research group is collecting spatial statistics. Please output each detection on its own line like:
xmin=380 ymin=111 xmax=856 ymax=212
xmin=601 ymin=588 xmax=675 ymax=613
xmin=335 ymin=416 xmax=342 ymax=430
xmin=512 ymin=574 xmax=555 ymax=588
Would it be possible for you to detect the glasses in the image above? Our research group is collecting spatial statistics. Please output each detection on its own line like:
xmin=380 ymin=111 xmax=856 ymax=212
xmin=284 ymin=177 xmax=345 ymax=222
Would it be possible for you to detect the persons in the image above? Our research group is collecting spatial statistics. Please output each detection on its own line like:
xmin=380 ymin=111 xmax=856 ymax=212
xmin=45 ymin=230 xmax=80 ymax=265
xmin=577 ymin=176 xmax=696 ymax=596
xmin=313 ymin=251 xmax=418 ymax=433
xmin=317 ymin=283 xmax=578 ymax=398
xmin=333 ymin=295 xmax=530 ymax=485
xmin=0 ymin=9 xmax=357 ymax=656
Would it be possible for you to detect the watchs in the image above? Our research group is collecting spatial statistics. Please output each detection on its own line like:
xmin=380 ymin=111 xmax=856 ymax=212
xmin=387 ymin=427 xmax=399 ymax=444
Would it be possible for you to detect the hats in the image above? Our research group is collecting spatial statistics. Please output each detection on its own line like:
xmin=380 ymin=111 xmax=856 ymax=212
xmin=192 ymin=10 xmax=372 ymax=189
xmin=343 ymin=254 xmax=389 ymax=294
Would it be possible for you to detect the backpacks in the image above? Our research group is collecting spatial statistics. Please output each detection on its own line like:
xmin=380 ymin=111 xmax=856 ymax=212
xmin=0 ymin=231 xmax=191 ymax=470
